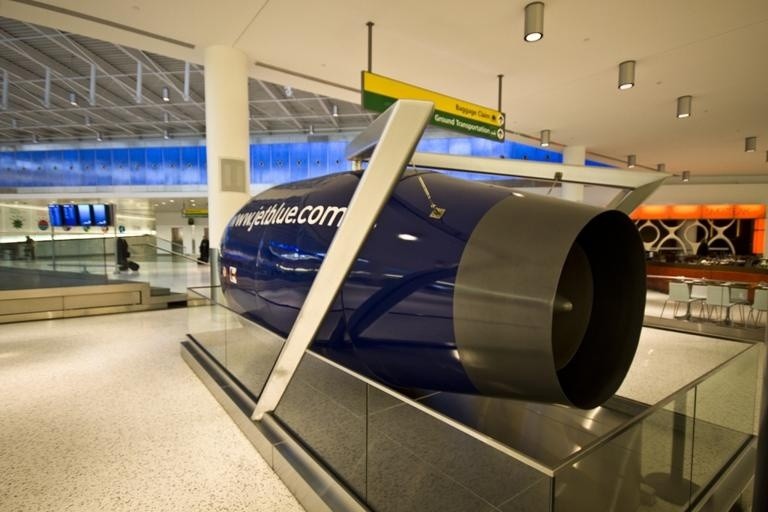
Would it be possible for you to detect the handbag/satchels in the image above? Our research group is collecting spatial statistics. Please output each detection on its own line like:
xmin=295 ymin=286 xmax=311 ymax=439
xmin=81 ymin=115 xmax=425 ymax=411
xmin=126 ymin=252 xmax=130 ymax=257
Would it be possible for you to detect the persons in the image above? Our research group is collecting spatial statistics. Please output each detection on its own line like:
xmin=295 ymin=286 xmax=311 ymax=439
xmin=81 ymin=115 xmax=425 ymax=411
xmin=696 ymin=240 xmax=709 ymax=255
xmin=200 ymin=235 xmax=209 ymax=262
xmin=116 ymin=237 xmax=129 ymax=272
xmin=24 ymin=235 xmax=36 ymax=260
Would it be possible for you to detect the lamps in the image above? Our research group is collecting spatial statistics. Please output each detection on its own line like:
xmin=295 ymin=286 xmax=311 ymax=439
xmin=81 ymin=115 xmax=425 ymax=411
xmin=523 ymin=2 xmax=545 ymax=45
xmin=539 ymin=130 xmax=551 ymax=146
xmin=616 ymin=61 xmax=756 ymax=182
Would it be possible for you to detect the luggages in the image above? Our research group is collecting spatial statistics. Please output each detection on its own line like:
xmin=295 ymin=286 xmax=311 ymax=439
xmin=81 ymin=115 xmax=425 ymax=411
xmin=128 ymin=261 xmax=139 ymax=271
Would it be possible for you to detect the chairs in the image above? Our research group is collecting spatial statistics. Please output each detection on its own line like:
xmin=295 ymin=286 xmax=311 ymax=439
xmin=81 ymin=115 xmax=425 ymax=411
xmin=659 ymin=282 xmax=768 ymax=329
